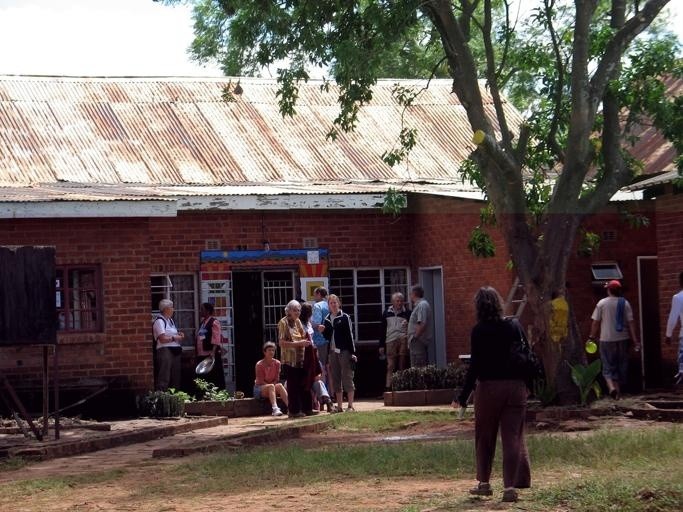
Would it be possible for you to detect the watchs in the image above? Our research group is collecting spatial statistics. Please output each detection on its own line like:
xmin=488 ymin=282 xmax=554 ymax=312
xmin=172 ymin=336 xmax=175 ymax=342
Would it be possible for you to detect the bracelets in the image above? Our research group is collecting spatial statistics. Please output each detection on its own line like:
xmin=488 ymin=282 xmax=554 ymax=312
xmin=588 ymin=336 xmax=594 ymax=340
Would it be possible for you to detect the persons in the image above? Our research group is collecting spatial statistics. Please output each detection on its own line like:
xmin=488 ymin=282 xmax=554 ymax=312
xmin=376 ymin=292 xmax=412 ymax=400
xmin=586 ymin=279 xmax=640 ymax=400
xmin=152 ymin=299 xmax=184 ymax=391
xmin=195 ymin=303 xmax=225 ymax=393
xmin=277 ymin=286 xmax=358 ymax=417
xmin=665 ymin=272 xmax=683 ymax=383
xmin=451 ymin=286 xmax=532 ymax=502
xmin=407 ymin=286 xmax=432 ymax=368
xmin=253 ymin=341 xmax=289 ymax=416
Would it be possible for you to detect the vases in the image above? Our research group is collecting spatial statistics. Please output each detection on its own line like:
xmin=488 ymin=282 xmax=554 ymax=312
xmin=146 ymin=387 xmax=197 ymax=417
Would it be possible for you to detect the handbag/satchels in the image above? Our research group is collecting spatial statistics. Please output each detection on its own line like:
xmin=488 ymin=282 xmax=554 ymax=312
xmin=520 ymin=340 xmax=547 ymax=401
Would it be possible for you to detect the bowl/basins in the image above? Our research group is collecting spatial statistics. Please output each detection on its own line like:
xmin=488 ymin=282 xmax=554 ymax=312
xmin=195 ymin=357 xmax=216 ymax=375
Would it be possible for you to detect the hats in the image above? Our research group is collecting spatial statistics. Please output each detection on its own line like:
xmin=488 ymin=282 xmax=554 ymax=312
xmin=602 ymin=280 xmax=622 ymax=289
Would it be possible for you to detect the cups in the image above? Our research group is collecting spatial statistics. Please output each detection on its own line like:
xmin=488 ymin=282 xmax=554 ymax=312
xmin=234 ymin=391 xmax=244 ymax=399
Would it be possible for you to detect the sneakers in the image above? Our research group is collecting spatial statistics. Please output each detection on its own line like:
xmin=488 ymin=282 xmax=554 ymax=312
xmin=270 ymin=403 xmax=357 ymax=418
xmin=467 ymin=481 xmax=493 ymax=497
xmin=499 ymin=489 xmax=519 ymax=503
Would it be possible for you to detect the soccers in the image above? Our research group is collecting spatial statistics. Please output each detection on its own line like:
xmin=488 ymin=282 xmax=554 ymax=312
xmin=585 ymin=342 xmax=597 ymax=354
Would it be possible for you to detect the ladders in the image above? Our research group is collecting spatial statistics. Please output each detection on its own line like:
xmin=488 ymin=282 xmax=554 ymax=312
xmin=458 ymin=277 xmax=528 ymax=422
